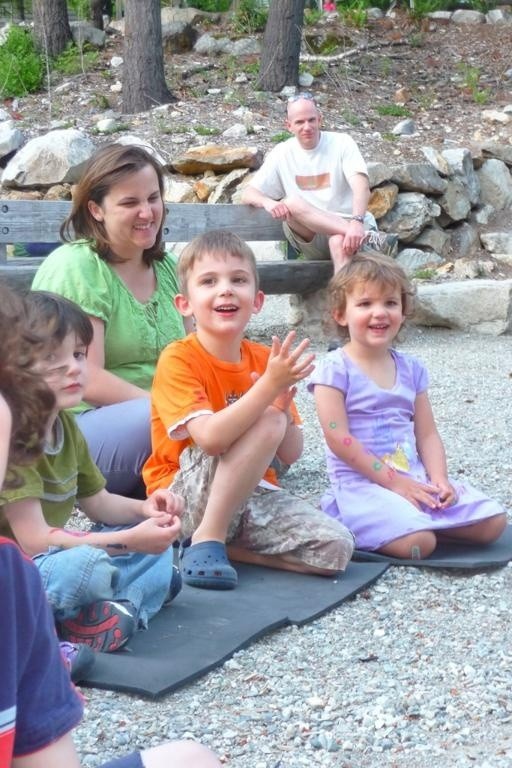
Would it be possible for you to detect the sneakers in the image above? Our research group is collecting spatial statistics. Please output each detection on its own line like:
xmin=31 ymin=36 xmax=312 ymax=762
xmin=163 ymin=563 xmax=183 ymax=606
xmin=57 ymin=600 xmax=137 ymax=683
xmin=359 ymin=230 xmax=400 ymax=259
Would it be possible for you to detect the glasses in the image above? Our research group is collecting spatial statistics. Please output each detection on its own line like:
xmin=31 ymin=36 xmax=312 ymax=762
xmin=285 ymin=92 xmax=316 ymax=114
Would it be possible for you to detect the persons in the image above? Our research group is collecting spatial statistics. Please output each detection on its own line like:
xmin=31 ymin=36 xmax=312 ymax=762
xmin=29 ymin=144 xmax=192 ymax=496
xmin=0 ymin=287 xmax=225 ymax=768
xmin=142 ymin=229 xmax=350 ymax=587
xmin=304 ymin=247 xmax=505 ymax=559
xmin=9 ymin=293 xmax=183 ymax=658
xmin=251 ymin=96 xmax=397 ymax=327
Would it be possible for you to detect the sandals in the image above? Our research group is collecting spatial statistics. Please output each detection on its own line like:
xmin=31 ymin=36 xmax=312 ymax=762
xmin=178 ymin=534 xmax=238 ymax=606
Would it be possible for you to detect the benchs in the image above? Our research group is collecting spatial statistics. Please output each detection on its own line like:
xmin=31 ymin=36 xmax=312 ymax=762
xmin=0 ymin=200 xmax=334 ymax=296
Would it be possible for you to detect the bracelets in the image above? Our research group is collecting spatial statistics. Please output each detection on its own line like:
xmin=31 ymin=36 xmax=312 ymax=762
xmin=348 ymin=212 xmax=368 ymax=224
